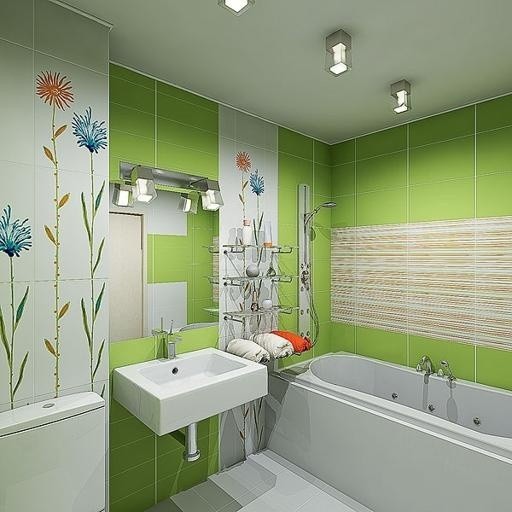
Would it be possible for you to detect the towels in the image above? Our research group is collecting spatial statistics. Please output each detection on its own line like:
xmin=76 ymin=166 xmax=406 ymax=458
xmin=226 ymin=330 xmax=311 ymax=363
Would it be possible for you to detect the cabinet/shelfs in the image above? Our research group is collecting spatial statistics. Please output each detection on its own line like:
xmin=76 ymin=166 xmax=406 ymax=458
xmin=222 ymin=243 xmax=303 ymax=365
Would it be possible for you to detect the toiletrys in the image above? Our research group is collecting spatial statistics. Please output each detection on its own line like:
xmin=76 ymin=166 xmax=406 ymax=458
xmin=264 ymin=220 xmax=273 ymax=248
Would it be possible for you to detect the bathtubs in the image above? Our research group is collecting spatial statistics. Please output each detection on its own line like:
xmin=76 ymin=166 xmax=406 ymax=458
xmin=280 ymin=352 xmax=512 ymax=461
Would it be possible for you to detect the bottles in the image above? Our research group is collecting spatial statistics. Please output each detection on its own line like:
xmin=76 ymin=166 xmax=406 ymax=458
xmin=243 ymin=219 xmax=253 ymax=245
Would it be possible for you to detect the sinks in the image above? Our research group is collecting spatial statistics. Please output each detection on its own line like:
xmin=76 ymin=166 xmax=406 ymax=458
xmin=114 ymin=347 xmax=268 ymax=437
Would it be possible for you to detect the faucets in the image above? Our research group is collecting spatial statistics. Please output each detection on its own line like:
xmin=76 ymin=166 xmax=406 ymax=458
xmin=167 ymin=320 xmax=181 ymax=359
xmin=414 ymin=355 xmax=433 ymax=375
xmin=150 ymin=317 xmax=166 ymax=336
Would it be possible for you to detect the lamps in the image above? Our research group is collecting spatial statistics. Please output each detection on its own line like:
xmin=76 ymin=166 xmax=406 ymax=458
xmin=113 ymin=182 xmax=191 ymax=214
xmin=120 ymin=161 xmax=225 ymax=213
xmin=220 ymin=0 xmax=413 ymax=115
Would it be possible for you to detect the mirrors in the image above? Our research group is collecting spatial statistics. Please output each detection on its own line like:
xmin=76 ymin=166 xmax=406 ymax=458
xmin=147 ymin=281 xmax=188 ymax=337
xmin=109 ymin=182 xmax=188 ymax=237
